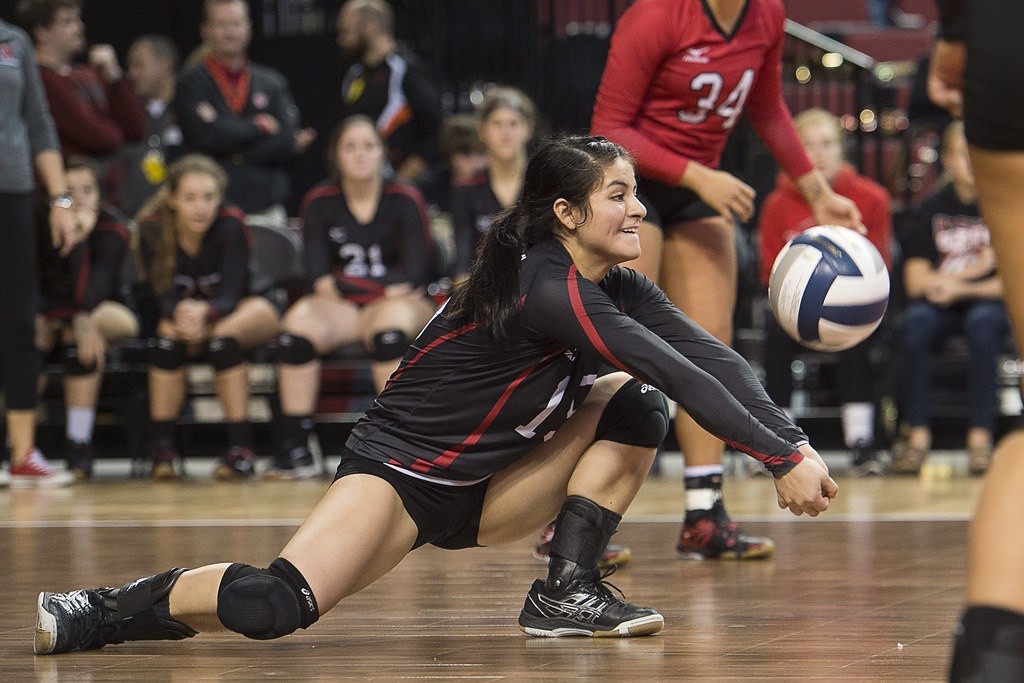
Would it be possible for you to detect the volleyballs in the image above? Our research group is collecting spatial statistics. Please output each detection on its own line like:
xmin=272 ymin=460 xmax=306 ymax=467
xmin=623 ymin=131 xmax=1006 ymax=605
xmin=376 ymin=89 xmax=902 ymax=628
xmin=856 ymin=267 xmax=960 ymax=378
xmin=767 ymin=224 xmax=891 ymax=353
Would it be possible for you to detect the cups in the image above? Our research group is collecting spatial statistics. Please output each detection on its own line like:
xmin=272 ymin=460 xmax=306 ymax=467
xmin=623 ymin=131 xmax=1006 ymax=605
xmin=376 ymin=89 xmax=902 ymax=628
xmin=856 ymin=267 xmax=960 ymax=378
xmin=843 ymin=401 xmax=874 ymax=447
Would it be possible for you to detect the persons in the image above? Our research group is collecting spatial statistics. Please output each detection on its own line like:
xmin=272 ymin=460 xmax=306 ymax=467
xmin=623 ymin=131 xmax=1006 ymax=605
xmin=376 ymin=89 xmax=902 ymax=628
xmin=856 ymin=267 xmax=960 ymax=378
xmin=0 ymin=0 xmax=1024 ymax=682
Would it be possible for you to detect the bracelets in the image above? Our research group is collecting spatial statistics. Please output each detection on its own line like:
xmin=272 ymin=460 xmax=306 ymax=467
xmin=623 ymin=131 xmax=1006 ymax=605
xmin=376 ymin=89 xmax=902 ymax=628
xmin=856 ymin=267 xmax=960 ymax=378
xmin=47 ymin=195 xmax=71 ymax=208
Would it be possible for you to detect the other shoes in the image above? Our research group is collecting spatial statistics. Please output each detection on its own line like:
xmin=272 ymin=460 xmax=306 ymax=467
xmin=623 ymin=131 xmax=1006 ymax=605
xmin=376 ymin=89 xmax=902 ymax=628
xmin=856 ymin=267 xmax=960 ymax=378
xmin=9 ymin=448 xmax=77 ymax=488
xmin=853 ymin=442 xmax=887 ymax=478
xmin=57 ymin=442 xmax=94 ymax=479
xmin=150 ymin=450 xmax=182 ymax=479
xmin=888 ymin=447 xmax=926 ymax=472
xmin=215 ymin=454 xmax=255 ymax=481
xmin=265 ymin=452 xmax=321 ymax=481
xmin=967 ymin=443 xmax=992 ymax=473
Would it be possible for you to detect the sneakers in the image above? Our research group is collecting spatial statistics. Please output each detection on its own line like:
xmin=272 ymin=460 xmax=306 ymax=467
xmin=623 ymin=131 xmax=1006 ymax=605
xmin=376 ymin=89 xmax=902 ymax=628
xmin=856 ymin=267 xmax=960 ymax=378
xmin=677 ymin=513 xmax=775 ymax=561
xmin=533 ymin=525 xmax=634 ymax=578
xmin=517 ymin=564 xmax=666 ymax=637
xmin=34 ymin=585 xmax=114 ymax=654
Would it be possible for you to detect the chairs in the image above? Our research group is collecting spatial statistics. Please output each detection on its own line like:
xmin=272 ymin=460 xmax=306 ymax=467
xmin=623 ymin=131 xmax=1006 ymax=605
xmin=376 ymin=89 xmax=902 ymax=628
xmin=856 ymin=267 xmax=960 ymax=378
xmin=249 ymin=222 xmax=295 ymax=310
